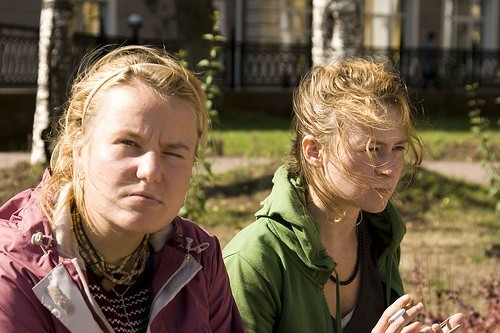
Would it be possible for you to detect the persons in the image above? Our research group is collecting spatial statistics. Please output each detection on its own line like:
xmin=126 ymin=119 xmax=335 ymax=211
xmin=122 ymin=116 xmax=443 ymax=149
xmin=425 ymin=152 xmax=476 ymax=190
xmin=423 ymin=32 xmax=440 ymax=83
xmin=227 ymin=57 xmax=465 ymax=333
xmin=0 ymin=43 xmax=250 ymax=333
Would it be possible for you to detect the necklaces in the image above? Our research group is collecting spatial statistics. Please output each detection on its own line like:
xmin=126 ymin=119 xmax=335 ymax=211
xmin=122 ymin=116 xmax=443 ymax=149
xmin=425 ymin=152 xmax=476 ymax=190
xmin=331 ymin=219 xmax=363 ymax=286
xmin=72 ymin=205 xmax=151 ymax=285
